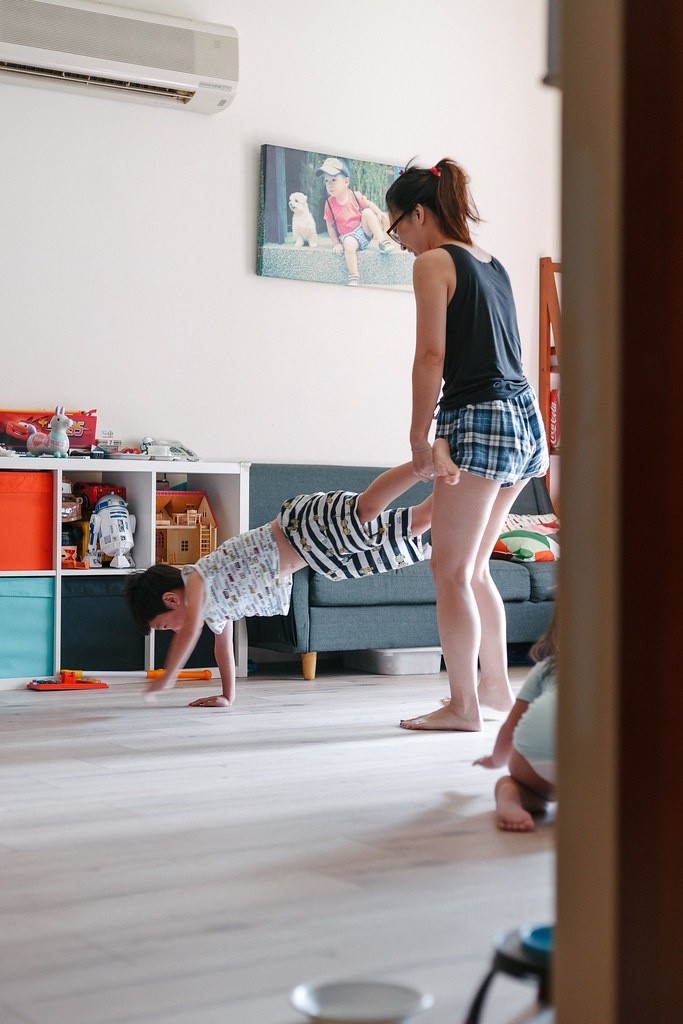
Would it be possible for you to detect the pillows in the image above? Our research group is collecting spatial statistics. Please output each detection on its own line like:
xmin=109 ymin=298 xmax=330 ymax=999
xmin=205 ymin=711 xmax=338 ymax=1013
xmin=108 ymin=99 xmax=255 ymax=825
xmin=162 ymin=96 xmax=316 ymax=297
xmin=489 ymin=512 xmax=561 ymax=563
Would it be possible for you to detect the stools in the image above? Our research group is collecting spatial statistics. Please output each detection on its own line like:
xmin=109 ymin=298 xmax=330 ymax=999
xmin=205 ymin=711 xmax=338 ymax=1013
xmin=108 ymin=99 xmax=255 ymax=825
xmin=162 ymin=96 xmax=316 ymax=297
xmin=461 ymin=925 xmax=557 ymax=1023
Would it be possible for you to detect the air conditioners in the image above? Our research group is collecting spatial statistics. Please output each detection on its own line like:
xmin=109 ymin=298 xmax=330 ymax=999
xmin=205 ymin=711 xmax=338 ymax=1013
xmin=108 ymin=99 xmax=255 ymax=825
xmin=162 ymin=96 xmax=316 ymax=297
xmin=0 ymin=0 xmax=240 ymax=117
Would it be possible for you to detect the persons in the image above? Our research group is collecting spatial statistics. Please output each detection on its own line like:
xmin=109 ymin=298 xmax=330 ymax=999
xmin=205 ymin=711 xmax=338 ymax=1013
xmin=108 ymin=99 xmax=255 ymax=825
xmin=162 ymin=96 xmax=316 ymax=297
xmin=122 ymin=438 xmax=460 ymax=707
xmin=469 ymin=600 xmax=560 ymax=836
xmin=314 ymin=157 xmax=394 ymax=285
xmin=384 ymin=155 xmax=549 ymax=733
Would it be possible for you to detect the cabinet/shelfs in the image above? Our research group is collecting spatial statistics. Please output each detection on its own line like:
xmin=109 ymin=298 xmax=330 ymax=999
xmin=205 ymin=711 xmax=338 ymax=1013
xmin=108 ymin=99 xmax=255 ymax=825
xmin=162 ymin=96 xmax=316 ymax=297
xmin=538 ymin=257 xmax=560 ymax=520
xmin=0 ymin=460 xmax=254 ymax=692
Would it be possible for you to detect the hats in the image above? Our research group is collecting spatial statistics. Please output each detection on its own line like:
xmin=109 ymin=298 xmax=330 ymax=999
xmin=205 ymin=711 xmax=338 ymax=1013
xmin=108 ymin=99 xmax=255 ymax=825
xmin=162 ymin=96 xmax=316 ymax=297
xmin=314 ymin=158 xmax=350 ymax=178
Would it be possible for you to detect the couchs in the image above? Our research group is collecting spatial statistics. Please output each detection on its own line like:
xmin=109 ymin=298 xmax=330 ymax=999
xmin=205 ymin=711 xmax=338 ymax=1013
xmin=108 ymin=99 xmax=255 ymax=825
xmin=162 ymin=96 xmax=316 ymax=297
xmin=243 ymin=463 xmax=565 ymax=681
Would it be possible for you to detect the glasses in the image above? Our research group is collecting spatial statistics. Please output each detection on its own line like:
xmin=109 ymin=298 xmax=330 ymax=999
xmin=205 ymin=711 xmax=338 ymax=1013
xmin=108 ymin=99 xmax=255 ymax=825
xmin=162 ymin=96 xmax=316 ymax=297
xmin=385 ymin=212 xmax=405 ymax=244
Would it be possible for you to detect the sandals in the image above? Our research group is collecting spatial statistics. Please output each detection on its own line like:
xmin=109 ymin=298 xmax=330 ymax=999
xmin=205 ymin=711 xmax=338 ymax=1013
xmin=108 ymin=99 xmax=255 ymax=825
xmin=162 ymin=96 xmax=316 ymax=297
xmin=347 ymin=275 xmax=359 ymax=288
xmin=378 ymin=239 xmax=398 ymax=255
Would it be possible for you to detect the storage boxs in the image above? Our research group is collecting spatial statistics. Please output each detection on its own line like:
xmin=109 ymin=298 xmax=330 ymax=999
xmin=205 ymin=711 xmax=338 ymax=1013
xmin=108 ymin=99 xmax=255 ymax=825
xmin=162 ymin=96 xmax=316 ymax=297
xmin=0 ymin=408 xmax=98 ymax=452
xmin=342 ymin=646 xmax=444 ymax=675
xmin=0 ymin=577 xmax=55 ymax=679
xmin=0 ymin=471 xmax=52 ymax=572
xmin=155 ymin=621 xmax=236 ymax=671
xmin=60 ymin=579 xmax=145 ymax=672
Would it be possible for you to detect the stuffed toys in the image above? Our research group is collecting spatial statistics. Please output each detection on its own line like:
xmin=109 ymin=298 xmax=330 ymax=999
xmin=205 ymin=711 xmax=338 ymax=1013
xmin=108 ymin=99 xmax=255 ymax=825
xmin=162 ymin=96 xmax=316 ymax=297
xmin=24 ymin=404 xmax=73 ymax=458
